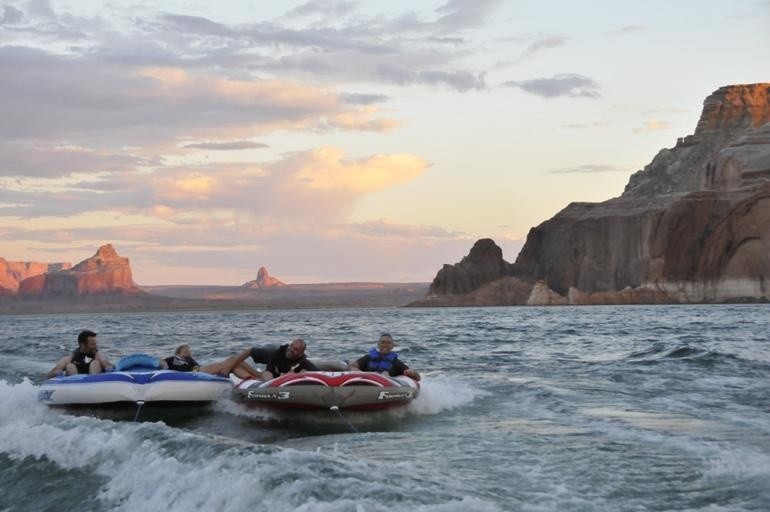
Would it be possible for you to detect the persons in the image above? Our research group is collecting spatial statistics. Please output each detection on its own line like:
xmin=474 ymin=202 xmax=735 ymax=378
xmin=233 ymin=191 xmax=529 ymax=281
xmin=160 ymin=344 xmax=273 ymax=380
xmin=47 ymin=331 xmax=116 ymax=380
xmin=224 ymin=338 xmax=319 ymax=378
xmin=348 ymin=334 xmax=421 ymax=381
